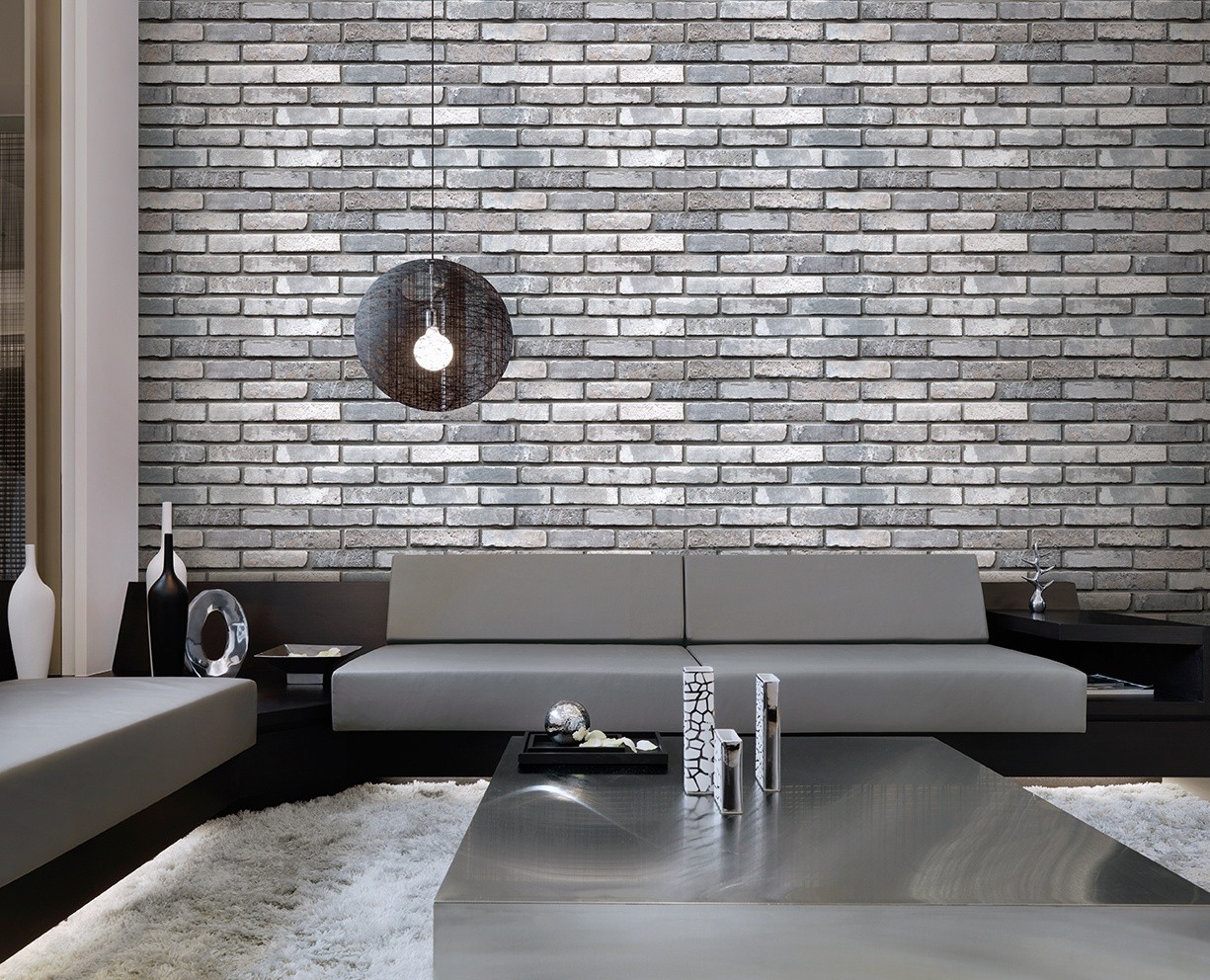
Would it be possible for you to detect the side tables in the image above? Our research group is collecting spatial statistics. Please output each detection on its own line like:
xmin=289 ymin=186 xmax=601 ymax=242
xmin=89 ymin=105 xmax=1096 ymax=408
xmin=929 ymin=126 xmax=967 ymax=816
xmin=986 ymin=608 xmax=1210 ymax=779
xmin=240 ymin=644 xmax=362 ymax=759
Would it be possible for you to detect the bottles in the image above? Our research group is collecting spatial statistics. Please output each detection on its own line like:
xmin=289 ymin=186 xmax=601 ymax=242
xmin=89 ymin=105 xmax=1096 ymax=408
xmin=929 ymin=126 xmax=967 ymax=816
xmin=145 ymin=502 xmax=189 ymax=676
xmin=7 ymin=544 xmax=55 ymax=679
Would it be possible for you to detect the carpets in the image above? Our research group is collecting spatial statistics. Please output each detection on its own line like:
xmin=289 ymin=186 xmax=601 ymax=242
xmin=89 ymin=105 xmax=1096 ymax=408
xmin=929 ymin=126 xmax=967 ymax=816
xmin=1 ymin=779 xmax=1208 ymax=980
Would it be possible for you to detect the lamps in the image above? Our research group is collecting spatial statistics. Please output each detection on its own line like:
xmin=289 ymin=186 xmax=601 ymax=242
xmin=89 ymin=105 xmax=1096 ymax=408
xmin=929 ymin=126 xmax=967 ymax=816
xmin=411 ymin=1 xmax=453 ymax=371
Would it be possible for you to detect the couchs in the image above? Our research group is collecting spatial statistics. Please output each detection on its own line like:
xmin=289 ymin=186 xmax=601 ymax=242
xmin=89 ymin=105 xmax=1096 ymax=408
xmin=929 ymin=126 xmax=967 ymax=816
xmin=332 ymin=554 xmax=1087 ymax=773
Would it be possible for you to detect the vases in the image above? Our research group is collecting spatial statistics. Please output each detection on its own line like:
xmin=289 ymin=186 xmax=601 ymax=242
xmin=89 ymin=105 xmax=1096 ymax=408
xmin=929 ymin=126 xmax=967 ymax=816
xmin=146 ymin=501 xmax=187 ymax=677
xmin=148 ymin=533 xmax=189 ymax=677
xmin=8 ymin=543 xmax=55 ymax=681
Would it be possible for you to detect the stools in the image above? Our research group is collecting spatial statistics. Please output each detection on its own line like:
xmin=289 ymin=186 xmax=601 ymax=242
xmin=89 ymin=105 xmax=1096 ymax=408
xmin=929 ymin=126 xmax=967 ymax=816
xmin=1 ymin=675 xmax=258 ymax=896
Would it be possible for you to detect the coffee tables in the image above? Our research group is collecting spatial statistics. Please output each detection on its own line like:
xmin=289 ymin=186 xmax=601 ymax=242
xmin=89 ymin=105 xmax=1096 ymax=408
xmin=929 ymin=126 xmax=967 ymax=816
xmin=429 ymin=733 xmax=1209 ymax=980
